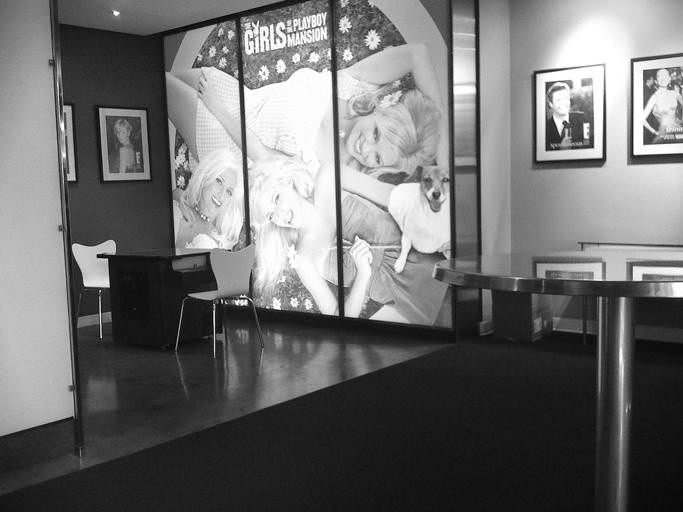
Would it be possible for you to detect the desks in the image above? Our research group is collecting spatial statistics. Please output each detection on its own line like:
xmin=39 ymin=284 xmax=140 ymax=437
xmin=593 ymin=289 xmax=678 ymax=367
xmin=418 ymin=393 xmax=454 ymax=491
xmin=433 ymin=252 xmax=683 ymax=512
xmin=97 ymin=248 xmax=223 ymax=350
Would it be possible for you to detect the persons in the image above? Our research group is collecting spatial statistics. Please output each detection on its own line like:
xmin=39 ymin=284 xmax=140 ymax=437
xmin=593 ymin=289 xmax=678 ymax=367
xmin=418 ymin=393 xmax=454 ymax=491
xmin=641 ymin=65 xmax=683 ymax=143
xmin=542 ymin=79 xmax=593 ymax=152
xmin=163 ymin=41 xmax=454 ymax=331
xmin=103 ymin=113 xmax=144 ymax=176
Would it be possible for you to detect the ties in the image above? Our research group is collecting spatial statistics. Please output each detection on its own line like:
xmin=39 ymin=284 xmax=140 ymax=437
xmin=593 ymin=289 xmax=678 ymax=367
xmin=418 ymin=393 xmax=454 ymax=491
xmin=561 ymin=120 xmax=568 ymax=142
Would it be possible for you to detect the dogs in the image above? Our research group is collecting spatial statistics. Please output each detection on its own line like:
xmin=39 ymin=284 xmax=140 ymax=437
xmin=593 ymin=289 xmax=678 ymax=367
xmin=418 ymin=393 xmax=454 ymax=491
xmin=388 ymin=164 xmax=451 ymax=274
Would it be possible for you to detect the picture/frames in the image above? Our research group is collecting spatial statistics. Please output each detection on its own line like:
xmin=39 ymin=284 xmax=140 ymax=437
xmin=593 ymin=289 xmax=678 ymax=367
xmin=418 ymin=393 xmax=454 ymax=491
xmin=625 ymin=260 xmax=683 ymax=344
xmin=532 ymin=257 xmax=606 ymax=338
xmin=63 ymin=103 xmax=78 ymax=184
xmin=532 ymin=63 xmax=606 ymax=163
xmin=96 ymin=105 xmax=153 ymax=183
xmin=630 ymin=52 xmax=683 ymax=158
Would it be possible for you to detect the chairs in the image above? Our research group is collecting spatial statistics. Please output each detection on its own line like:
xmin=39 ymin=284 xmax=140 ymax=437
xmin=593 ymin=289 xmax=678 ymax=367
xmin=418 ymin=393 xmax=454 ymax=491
xmin=71 ymin=240 xmax=117 ymax=339
xmin=174 ymin=243 xmax=264 ymax=357
